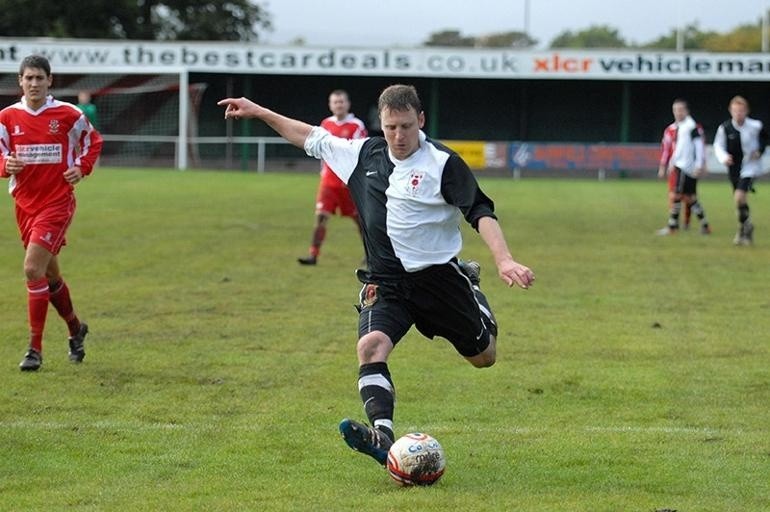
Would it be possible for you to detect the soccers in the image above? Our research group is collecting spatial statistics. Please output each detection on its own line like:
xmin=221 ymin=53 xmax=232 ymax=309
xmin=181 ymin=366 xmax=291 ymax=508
xmin=387 ymin=434 xmax=446 ymax=487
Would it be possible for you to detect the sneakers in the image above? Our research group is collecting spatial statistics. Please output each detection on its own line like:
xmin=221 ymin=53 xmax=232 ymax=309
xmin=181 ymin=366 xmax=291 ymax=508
xmin=339 ymin=418 xmax=395 ymax=469
xmin=299 ymin=258 xmax=316 ymax=264
xmin=67 ymin=324 xmax=88 ymax=362
xmin=734 ymin=223 xmax=753 ymax=246
xmin=20 ymin=349 xmax=41 ymax=369
xmin=459 ymin=261 xmax=481 ymax=280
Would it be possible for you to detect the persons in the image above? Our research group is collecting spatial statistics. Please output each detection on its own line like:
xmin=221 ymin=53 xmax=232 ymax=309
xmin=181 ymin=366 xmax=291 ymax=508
xmin=297 ymin=89 xmax=369 ymax=265
xmin=657 ymin=97 xmax=705 ymax=233
xmin=0 ymin=54 xmax=105 ymax=371
xmin=216 ymin=85 xmax=537 ymax=469
xmin=712 ymin=95 xmax=769 ymax=245
xmin=654 ymin=99 xmax=714 ymax=236
xmin=75 ymin=90 xmax=101 ymax=132
